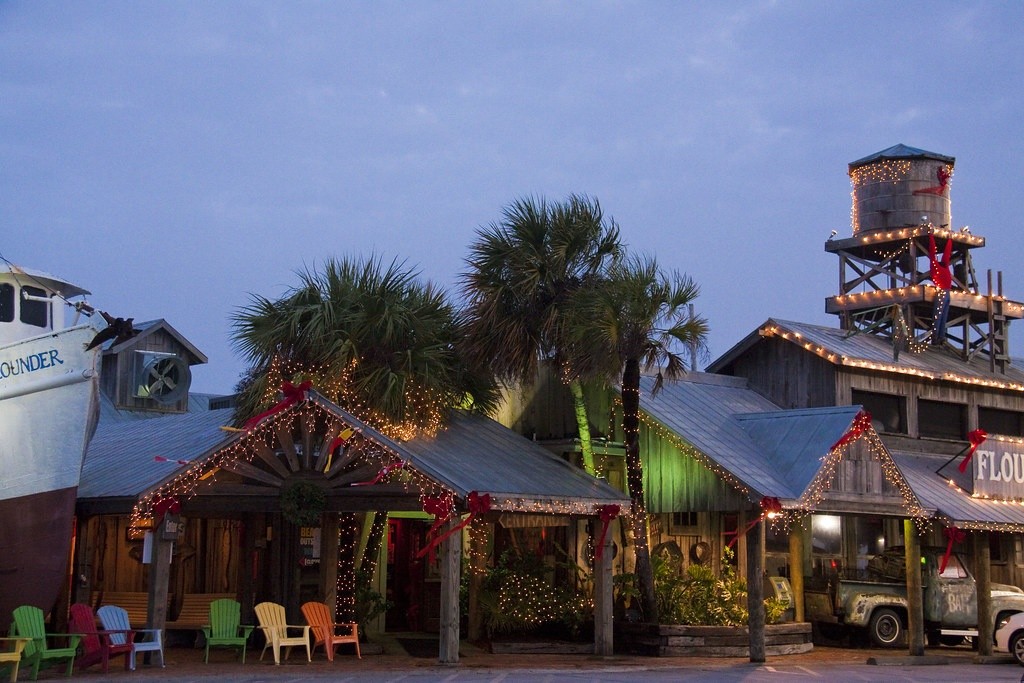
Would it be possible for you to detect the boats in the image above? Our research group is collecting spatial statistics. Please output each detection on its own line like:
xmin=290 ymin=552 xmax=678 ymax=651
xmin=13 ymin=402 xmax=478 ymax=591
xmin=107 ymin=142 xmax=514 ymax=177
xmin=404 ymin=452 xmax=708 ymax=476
xmin=0 ymin=252 xmax=104 ymax=648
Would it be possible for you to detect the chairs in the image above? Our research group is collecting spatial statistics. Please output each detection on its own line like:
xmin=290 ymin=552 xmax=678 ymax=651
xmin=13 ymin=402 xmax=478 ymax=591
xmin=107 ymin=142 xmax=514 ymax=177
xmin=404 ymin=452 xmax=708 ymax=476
xmin=97 ymin=605 xmax=165 ymax=671
xmin=201 ymin=599 xmax=255 ymax=665
xmin=0 ymin=638 xmax=33 ymax=683
xmin=57 ymin=604 xmax=136 ymax=674
xmin=254 ymin=602 xmax=311 ymax=665
xmin=301 ymin=602 xmax=361 ymax=662
xmin=0 ymin=606 xmax=87 ymax=682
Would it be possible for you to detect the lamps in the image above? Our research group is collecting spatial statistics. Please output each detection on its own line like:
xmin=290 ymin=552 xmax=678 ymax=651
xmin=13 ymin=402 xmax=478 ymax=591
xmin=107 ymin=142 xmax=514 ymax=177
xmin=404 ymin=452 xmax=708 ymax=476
xmin=921 ymin=215 xmax=928 ymax=225
xmin=935 ymin=429 xmax=988 ymax=473
xmin=828 ymin=229 xmax=838 ymax=241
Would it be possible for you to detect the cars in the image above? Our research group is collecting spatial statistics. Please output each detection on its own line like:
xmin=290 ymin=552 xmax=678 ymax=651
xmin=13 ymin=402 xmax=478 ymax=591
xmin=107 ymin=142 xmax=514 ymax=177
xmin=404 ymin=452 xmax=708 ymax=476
xmin=994 ymin=613 xmax=1024 ymax=664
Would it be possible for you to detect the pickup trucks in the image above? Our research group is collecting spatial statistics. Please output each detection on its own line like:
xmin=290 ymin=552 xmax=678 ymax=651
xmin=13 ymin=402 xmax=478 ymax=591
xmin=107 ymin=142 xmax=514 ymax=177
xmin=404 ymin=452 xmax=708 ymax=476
xmin=803 ymin=543 xmax=1024 ymax=649
